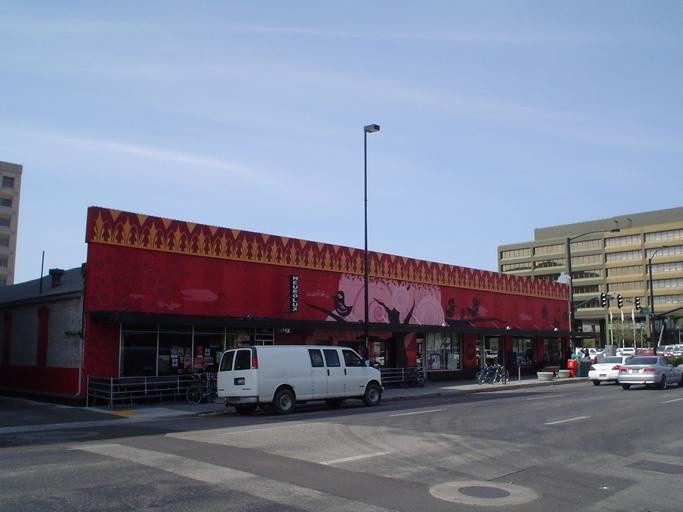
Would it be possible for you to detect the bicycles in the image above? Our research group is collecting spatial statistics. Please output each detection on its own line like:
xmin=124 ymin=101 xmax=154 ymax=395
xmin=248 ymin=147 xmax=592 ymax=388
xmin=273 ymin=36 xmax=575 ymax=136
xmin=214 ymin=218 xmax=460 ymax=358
xmin=476 ymin=364 xmax=509 ymax=385
xmin=185 ymin=374 xmax=226 ymax=405
xmin=415 ymin=365 xmax=425 ymax=386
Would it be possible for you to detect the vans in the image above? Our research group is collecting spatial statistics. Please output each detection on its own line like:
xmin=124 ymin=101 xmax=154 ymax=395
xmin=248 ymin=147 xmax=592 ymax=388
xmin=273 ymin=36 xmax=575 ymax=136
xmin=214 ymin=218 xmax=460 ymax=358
xmin=218 ymin=344 xmax=384 ymax=413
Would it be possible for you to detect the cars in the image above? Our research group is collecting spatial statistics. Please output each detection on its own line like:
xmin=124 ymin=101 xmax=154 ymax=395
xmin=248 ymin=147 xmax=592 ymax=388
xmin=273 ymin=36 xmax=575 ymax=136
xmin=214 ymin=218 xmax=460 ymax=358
xmin=588 ymin=356 xmax=629 ymax=386
xmin=576 ymin=343 xmax=683 ymax=358
xmin=619 ymin=356 xmax=683 ymax=390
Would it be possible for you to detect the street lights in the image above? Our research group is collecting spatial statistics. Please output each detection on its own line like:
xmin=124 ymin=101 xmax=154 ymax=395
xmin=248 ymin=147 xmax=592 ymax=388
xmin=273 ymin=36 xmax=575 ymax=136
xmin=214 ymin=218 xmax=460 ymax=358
xmin=364 ymin=123 xmax=380 ymax=344
xmin=648 ymin=244 xmax=668 ymax=355
xmin=565 ymin=227 xmax=620 ymax=353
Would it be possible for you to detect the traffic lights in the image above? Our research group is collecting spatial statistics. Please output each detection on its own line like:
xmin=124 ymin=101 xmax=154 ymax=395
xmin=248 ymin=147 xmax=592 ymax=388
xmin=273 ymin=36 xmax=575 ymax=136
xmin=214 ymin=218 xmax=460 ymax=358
xmin=618 ymin=295 xmax=623 ymax=308
xmin=601 ymin=294 xmax=607 ymax=307
xmin=635 ymin=297 xmax=639 ymax=309
